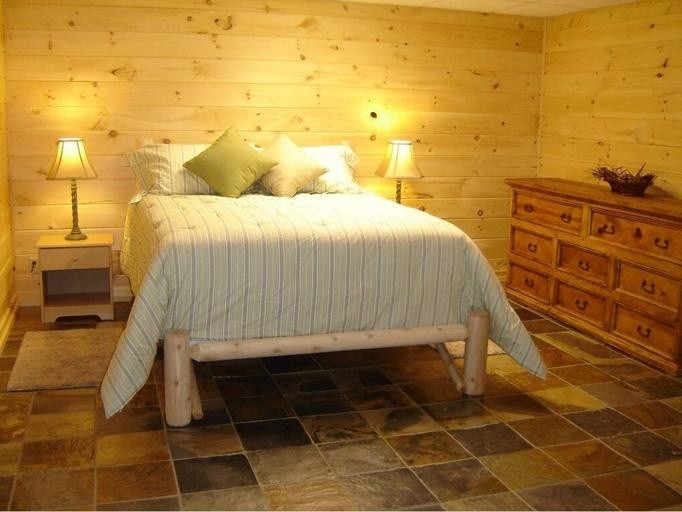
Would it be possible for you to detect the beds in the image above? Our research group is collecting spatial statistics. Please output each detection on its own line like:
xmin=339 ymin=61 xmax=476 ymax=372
xmin=120 ymin=136 xmax=490 ymax=427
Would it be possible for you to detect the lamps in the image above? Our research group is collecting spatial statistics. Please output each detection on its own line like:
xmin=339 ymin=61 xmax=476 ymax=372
xmin=374 ymin=140 xmax=424 ymax=205
xmin=45 ymin=137 xmax=97 ymax=241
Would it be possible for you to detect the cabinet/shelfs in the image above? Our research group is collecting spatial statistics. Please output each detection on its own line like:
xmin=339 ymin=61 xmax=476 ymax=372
xmin=503 ymin=178 xmax=682 ymax=377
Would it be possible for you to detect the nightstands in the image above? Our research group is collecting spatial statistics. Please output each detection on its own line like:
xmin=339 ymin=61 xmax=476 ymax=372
xmin=36 ymin=234 xmax=115 ymax=324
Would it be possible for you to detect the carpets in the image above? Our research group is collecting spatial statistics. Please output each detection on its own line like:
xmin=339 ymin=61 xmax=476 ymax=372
xmin=5 ymin=325 xmax=125 ymax=393
xmin=428 ymin=339 xmax=507 ymax=359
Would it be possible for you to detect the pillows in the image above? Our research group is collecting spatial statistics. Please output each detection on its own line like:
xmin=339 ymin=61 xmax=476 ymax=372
xmin=126 ymin=124 xmax=367 ymax=205
